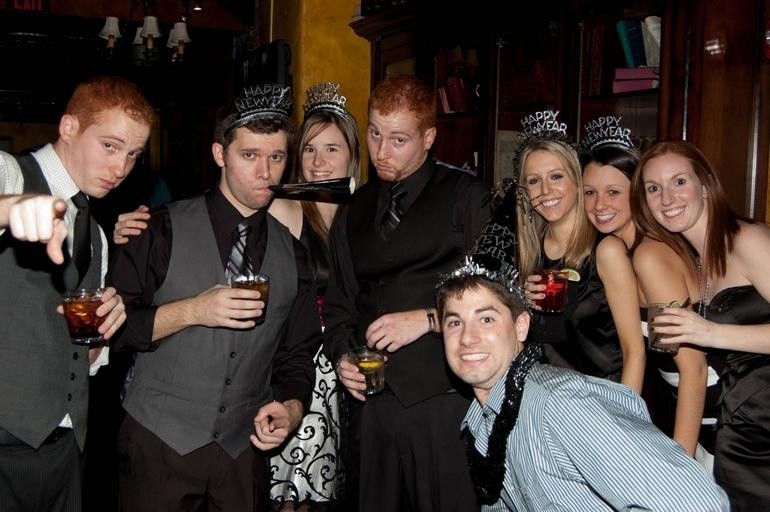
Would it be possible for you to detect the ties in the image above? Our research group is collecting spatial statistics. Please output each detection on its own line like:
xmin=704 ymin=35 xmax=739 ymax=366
xmin=379 ymin=181 xmax=404 ymax=240
xmin=225 ymin=220 xmax=254 ymax=287
xmin=71 ymin=190 xmax=92 ymax=286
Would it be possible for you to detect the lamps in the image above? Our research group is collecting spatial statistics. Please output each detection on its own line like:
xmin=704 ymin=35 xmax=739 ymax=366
xmin=172 ymin=23 xmax=191 ymax=61
xmin=167 ymin=29 xmax=179 ymax=63
xmin=134 ymin=27 xmax=146 ymax=44
xmin=101 ymin=17 xmax=121 ymax=56
xmin=98 ymin=24 xmax=117 ymax=60
xmin=140 ymin=16 xmax=161 ymax=57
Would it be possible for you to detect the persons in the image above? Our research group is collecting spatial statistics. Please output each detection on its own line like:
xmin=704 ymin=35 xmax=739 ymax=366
xmin=107 ymin=84 xmax=321 ymax=512
xmin=515 ymin=110 xmax=645 ymax=395
xmin=577 ymin=116 xmax=721 ymax=460
xmin=324 ymin=72 xmax=493 ymax=512
xmin=436 ymin=269 xmax=731 ymax=512
xmin=266 ymin=83 xmax=360 ymax=512
xmin=630 ymin=140 xmax=769 ymax=512
xmin=0 ymin=82 xmax=158 ymax=512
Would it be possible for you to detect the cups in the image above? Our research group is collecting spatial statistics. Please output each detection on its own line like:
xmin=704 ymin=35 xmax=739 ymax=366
xmin=645 ymin=302 xmax=682 ymax=351
xmin=232 ymin=275 xmax=268 ymax=326
xmin=350 ymin=347 xmax=392 ymax=393
xmin=61 ymin=288 xmax=106 ymax=344
xmin=535 ymin=271 xmax=568 ymax=311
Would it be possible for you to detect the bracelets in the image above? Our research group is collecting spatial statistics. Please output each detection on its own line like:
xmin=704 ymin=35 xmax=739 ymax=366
xmin=426 ymin=307 xmax=437 ymax=334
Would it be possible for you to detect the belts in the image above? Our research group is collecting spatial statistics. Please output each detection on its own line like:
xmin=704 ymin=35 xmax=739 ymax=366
xmin=47 ymin=427 xmax=73 ymax=443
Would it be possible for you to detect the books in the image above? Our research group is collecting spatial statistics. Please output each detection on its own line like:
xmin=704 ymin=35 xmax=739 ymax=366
xmin=439 ymin=79 xmax=473 ymax=114
xmin=613 ymin=16 xmax=661 ymax=94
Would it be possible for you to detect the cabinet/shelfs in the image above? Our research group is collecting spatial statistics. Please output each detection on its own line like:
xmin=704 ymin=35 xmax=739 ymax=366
xmin=349 ymin=1 xmax=703 ymax=223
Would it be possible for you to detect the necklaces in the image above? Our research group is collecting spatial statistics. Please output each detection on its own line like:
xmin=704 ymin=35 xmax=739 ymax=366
xmin=696 ymin=255 xmax=713 ymax=321
